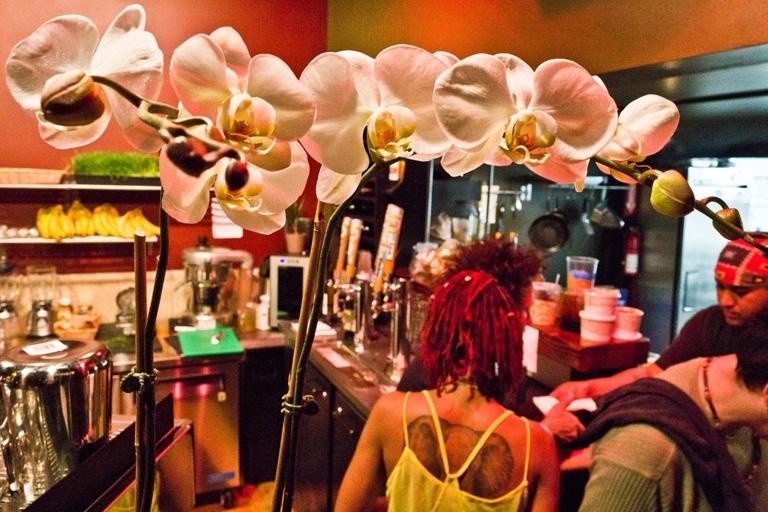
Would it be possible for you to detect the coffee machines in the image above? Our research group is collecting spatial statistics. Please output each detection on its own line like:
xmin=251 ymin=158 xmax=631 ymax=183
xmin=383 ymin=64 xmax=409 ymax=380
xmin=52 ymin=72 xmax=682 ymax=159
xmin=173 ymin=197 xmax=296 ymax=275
xmin=175 ymin=235 xmax=257 ymax=336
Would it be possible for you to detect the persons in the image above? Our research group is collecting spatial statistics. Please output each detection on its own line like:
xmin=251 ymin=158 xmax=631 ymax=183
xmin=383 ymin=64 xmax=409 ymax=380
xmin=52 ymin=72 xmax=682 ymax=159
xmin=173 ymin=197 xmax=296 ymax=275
xmin=548 ymin=233 xmax=768 ymax=413
xmin=576 ymin=307 xmax=768 ymax=512
xmin=397 ymin=234 xmax=587 ymax=443
xmin=333 ymin=269 xmax=561 ymax=512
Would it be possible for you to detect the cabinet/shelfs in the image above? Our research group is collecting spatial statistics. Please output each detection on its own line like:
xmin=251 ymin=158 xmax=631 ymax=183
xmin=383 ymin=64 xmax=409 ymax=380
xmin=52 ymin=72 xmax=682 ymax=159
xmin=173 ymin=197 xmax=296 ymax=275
xmin=1 ymin=167 xmax=161 ymax=244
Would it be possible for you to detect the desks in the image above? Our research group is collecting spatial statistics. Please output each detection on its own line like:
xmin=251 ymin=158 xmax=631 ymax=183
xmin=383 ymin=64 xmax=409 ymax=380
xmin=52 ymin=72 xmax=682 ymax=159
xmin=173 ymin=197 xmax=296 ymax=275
xmin=525 ymin=323 xmax=648 ymax=392
xmin=284 ymin=329 xmax=393 ymax=512
xmin=0 ymin=324 xmax=284 ymax=497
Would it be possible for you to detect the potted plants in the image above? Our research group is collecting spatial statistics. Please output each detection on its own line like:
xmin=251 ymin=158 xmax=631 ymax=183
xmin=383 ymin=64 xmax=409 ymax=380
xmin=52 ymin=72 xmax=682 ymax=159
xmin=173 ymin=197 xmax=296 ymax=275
xmin=284 ymin=203 xmax=311 ymax=254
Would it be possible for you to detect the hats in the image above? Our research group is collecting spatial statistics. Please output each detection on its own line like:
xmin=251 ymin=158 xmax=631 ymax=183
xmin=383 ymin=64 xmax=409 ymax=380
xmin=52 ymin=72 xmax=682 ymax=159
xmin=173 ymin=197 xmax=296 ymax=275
xmin=713 ymin=230 xmax=768 ymax=289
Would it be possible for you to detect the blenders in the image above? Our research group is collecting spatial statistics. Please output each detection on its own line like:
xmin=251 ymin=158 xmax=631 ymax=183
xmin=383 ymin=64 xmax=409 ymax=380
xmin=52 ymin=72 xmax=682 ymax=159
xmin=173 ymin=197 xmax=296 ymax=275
xmin=25 ymin=262 xmax=59 ymax=339
xmin=0 ymin=274 xmax=23 ymax=343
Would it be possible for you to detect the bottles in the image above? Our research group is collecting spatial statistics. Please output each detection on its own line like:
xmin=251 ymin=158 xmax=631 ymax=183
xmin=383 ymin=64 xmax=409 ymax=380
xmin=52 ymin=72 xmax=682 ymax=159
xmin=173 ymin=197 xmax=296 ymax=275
xmin=256 ymin=294 xmax=271 ymax=331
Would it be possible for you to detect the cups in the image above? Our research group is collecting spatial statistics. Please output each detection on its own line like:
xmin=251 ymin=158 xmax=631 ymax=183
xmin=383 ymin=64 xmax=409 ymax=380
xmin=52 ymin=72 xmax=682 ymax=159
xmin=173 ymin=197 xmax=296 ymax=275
xmin=285 ymin=219 xmax=312 ymax=256
xmin=530 ymin=255 xmax=644 ymax=343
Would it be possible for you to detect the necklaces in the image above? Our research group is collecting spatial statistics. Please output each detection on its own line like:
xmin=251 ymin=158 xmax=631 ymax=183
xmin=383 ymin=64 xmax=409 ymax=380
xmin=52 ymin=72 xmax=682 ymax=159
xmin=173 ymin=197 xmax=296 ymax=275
xmin=702 ymin=355 xmax=722 ymax=429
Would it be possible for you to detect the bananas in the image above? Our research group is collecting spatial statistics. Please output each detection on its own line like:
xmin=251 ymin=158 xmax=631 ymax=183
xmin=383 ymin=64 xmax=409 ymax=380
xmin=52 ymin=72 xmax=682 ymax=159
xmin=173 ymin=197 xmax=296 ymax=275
xmin=36 ymin=200 xmax=160 ymax=241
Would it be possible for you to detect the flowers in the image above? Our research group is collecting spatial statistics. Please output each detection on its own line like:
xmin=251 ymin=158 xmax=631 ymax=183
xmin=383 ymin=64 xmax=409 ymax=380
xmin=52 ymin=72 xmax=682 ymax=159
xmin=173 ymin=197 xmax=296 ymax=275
xmin=5 ymin=4 xmax=767 ymax=512
